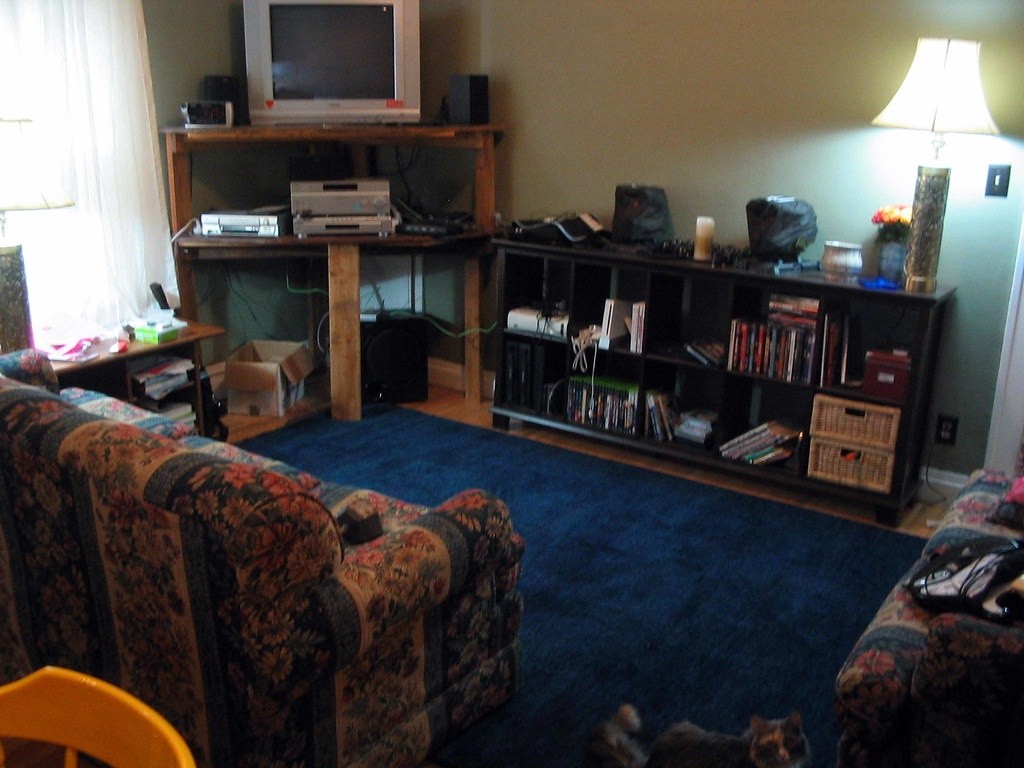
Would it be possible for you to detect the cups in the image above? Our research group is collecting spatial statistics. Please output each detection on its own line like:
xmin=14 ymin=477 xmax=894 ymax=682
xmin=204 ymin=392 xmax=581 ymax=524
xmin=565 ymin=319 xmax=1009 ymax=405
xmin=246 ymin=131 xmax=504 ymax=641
xmin=694 ymin=216 xmax=715 ymax=261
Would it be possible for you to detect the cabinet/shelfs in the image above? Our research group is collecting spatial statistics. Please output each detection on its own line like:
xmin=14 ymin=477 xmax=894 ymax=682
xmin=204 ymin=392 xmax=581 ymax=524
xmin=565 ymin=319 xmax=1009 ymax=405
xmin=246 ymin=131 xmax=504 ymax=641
xmin=160 ymin=122 xmax=504 ymax=423
xmin=489 ymin=233 xmax=959 ymax=528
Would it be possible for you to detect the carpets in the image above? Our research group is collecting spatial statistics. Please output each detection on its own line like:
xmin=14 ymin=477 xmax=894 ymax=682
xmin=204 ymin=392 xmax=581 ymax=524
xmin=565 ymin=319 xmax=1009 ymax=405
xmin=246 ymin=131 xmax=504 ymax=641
xmin=226 ymin=401 xmax=930 ymax=768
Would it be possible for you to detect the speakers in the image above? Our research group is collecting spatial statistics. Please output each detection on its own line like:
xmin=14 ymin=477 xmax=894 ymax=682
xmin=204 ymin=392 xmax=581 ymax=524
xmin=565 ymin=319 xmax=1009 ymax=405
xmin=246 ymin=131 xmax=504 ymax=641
xmin=448 ymin=74 xmax=490 ymax=125
xmin=205 ymin=74 xmax=239 ymax=126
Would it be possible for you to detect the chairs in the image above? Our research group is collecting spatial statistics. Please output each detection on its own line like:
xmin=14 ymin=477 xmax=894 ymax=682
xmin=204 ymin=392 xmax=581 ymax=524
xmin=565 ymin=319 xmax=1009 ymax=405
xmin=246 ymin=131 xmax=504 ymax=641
xmin=0 ymin=665 xmax=196 ymax=768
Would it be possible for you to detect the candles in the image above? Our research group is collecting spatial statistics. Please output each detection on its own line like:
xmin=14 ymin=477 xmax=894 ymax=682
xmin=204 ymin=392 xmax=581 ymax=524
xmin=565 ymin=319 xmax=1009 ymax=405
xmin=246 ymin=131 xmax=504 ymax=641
xmin=694 ymin=217 xmax=716 ymax=263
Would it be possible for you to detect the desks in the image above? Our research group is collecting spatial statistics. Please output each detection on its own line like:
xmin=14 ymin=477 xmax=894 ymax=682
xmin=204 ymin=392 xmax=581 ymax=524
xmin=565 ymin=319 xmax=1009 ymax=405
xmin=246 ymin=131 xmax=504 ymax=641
xmin=46 ymin=319 xmax=225 ymax=438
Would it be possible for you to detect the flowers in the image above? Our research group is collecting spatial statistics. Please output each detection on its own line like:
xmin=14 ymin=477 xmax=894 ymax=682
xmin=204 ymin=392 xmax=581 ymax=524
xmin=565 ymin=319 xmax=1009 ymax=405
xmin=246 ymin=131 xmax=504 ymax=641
xmin=871 ymin=204 xmax=913 ymax=246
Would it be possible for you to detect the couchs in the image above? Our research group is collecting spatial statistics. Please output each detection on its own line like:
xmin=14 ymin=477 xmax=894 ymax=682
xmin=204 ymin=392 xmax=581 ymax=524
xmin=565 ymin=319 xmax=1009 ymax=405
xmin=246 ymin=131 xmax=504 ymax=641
xmin=829 ymin=442 xmax=1024 ymax=768
xmin=0 ymin=348 xmax=526 ymax=768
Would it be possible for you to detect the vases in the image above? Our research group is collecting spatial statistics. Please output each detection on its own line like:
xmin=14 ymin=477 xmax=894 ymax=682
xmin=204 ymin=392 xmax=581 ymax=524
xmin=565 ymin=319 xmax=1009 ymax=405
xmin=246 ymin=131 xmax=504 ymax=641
xmin=820 ymin=240 xmax=863 ymax=284
xmin=878 ymin=241 xmax=906 ymax=282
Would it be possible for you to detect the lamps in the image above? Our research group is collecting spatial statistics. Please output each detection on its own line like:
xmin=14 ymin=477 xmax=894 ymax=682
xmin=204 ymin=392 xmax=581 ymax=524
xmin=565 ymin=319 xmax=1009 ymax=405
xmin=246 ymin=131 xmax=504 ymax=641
xmin=871 ymin=36 xmax=1006 ymax=297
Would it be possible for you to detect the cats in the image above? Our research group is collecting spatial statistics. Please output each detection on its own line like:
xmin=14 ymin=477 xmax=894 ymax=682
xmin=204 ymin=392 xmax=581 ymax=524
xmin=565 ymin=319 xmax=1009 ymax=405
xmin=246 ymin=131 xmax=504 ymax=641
xmin=597 ymin=703 xmax=815 ymax=768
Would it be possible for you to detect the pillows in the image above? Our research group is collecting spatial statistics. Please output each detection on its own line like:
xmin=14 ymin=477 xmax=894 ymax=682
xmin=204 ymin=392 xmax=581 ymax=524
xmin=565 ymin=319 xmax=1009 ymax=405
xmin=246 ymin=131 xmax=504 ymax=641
xmin=901 ymin=534 xmax=1024 ymax=626
xmin=984 ymin=473 xmax=1024 ymax=531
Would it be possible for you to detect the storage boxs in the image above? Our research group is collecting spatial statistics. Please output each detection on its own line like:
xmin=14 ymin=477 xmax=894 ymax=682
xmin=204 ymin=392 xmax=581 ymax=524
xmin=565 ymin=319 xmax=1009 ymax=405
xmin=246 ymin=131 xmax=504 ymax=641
xmin=224 ymin=340 xmax=317 ymax=418
xmin=428 ymin=326 xmax=495 ymax=400
xmin=134 ymin=327 xmax=179 ymax=344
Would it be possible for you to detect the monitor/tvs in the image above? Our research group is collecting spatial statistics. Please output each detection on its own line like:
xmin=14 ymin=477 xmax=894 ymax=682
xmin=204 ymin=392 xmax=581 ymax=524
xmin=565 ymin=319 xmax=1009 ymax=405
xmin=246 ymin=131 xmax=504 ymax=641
xmin=244 ymin=0 xmax=420 ymax=126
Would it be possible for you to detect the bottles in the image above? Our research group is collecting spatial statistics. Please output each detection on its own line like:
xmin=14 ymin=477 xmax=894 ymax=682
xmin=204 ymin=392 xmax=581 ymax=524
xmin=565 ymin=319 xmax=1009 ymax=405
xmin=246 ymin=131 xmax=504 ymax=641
xmin=822 ymin=241 xmax=863 ymax=283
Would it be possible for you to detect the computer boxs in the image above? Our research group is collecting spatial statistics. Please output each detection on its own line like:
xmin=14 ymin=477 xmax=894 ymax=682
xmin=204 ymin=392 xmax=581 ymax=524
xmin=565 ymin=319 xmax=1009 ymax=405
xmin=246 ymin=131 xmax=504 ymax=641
xmin=361 ymin=318 xmax=428 ymax=403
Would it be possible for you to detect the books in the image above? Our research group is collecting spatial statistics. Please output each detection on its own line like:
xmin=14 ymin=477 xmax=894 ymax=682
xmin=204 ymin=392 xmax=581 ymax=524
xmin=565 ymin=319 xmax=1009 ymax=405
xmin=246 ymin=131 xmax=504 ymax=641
xmin=128 ymin=354 xmax=195 ymax=400
xmin=716 ymin=420 xmax=802 ymax=468
xmin=501 ymin=336 xmax=718 ymax=447
xmin=684 ymin=293 xmax=856 ymax=391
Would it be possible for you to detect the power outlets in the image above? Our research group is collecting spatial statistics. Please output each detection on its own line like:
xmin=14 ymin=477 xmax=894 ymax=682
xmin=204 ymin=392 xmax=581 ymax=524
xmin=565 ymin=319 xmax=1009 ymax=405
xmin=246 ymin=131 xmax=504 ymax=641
xmin=934 ymin=415 xmax=959 ymax=447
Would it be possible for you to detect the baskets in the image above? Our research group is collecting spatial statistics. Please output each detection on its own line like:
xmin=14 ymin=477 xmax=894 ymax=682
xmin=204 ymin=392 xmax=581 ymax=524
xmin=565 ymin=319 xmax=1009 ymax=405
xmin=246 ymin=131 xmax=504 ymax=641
xmin=806 ymin=437 xmax=896 ymax=495
xmin=809 ymin=395 xmax=901 ymax=454
